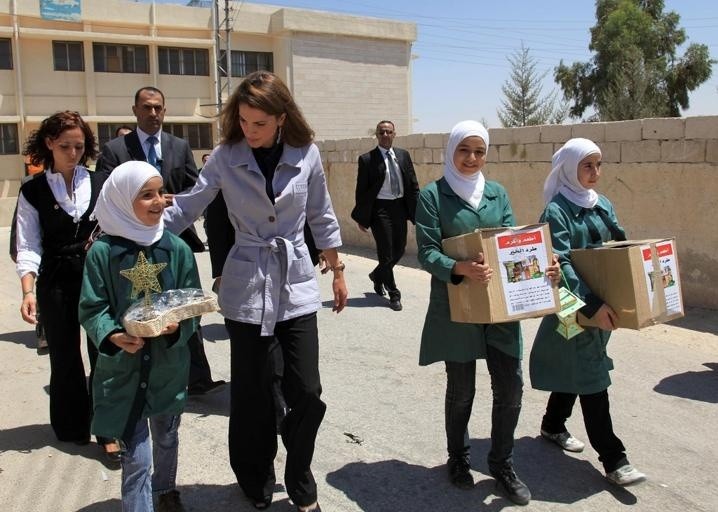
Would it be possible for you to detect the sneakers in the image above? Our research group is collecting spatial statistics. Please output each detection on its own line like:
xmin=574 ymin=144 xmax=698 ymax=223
xmin=606 ymin=464 xmax=646 ymax=485
xmin=447 ymin=458 xmax=475 ymax=491
xmin=157 ymin=489 xmax=184 ymax=512
xmin=36 ymin=335 xmax=49 ymax=349
xmin=540 ymin=428 xmax=585 ymax=451
xmin=488 ymin=461 xmax=532 ymax=505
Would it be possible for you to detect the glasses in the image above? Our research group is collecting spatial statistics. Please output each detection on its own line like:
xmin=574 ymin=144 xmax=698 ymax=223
xmin=380 ymin=129 xmax=394 ymax=135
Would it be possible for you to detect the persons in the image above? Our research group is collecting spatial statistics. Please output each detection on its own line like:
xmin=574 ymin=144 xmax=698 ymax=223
xmin=9 ymin=167 xmax=50 ymax=355
xmin=116 ymin=126 xmax=134 ymax=138
xmin=529 ymin=136 xmax=645 ymax=486
xmin=203 ymin=186 xmax=332 ymax=434
xmin=351 ymin=121 xmax=420 ymax=311
xmin=163 ymin=69 xmax=350 ymax=511
xmin=414 ymin=119 xmax=561 ymax=507
xmin=78 ymin=159 xmax=206 ymax=512
xmin=16 ymin=110 xmax=124 ymax=465
xmin=198 ymin=154 xmax=211 ymax=176
xmin=97 ymin=87 xmax=227 ymax=398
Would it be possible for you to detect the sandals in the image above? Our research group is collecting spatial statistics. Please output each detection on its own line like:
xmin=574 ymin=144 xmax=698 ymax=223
xmin=252 ymin=492 xmax=272 ymax=509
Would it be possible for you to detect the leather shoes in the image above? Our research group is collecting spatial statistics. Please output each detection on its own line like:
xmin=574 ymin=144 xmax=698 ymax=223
xmin=389 ymin=299 xmax=402 ymax=310
xmin=368 ymin=271 xmax=386 ymax=295
xmin=98 ymin=442 xmax=122 ymax=461
xmin=187 ymin=377 xmax=224 ymax=395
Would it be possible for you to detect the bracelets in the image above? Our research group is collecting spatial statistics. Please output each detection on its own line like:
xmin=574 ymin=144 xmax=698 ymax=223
xmin=330 ymin=261 xmax=345 ymax=276
xmin=23 ymin=290 xmax=34 ymax=299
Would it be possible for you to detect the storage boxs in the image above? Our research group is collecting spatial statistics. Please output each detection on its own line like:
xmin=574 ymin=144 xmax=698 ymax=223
xmin=440 ymin=222 xmax=561 ymax=325
xmin=568 ymin=237 xmax=685 ymax=330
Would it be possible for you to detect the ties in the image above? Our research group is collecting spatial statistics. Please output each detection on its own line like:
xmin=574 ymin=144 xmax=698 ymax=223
xmin=385 ymin=151 xmax=401 ymax=198
xmin=146 ymin=137 xmax=159 ymax=168
